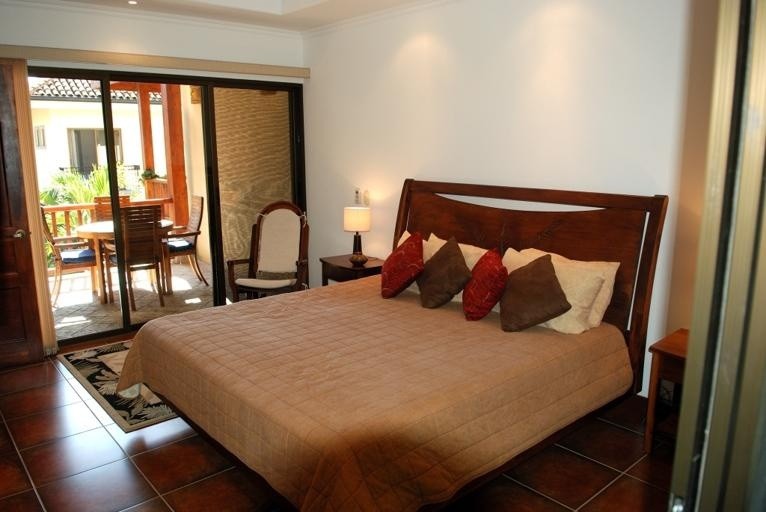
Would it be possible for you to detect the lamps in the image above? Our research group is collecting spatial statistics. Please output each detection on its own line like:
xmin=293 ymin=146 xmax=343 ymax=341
xmin=342 ymin=207 xmax=372 ymax=263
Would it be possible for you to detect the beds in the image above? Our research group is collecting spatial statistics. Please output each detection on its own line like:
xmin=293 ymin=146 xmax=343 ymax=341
xmin=115 ymin=179 xmax=669 ymax=507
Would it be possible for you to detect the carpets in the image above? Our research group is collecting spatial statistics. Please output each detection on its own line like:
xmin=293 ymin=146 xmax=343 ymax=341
xmin=56 ymin=338 xmax=180 ymax=432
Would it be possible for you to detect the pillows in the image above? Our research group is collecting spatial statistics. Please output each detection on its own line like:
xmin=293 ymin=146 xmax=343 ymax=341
xmin=381 ymin=230 xmax=622 ymax=334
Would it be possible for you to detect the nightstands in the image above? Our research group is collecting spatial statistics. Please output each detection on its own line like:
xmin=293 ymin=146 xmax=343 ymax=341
xmin=318 ymin=254 xmax=386 ymax=285
xmin=645 ymin=325 xmax=690 ymax=467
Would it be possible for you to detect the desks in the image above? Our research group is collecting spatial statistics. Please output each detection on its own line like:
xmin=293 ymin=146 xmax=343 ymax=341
xmin=77 ymin=218 xmax=173 ymax=303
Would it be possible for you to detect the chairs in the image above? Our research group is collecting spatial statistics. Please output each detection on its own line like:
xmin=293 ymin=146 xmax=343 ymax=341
xmin=148 ymin=193 xmax=209 ymax=292
xmin=40 ymin=206 xmax=112 ymax=305
xmin=226 ymin=199 xmax=310 ymax=304
xmin=92 ymin=194 xmax=134 ymax=286
xmin=104 ymin=203 xmax=165 ymax=312
